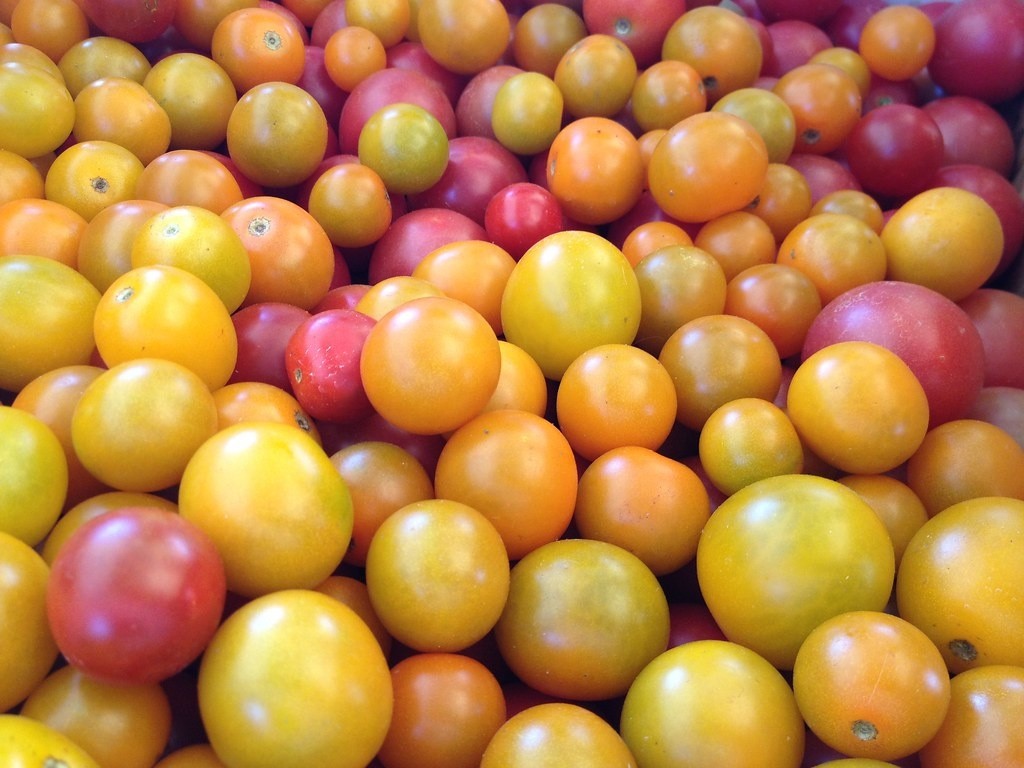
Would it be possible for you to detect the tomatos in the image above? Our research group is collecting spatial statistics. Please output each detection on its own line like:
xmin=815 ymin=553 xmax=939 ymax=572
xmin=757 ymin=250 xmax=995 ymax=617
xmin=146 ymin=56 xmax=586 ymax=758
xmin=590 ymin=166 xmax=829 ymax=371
xmin=0 ymin=0 xmax=1023 ymax=767
